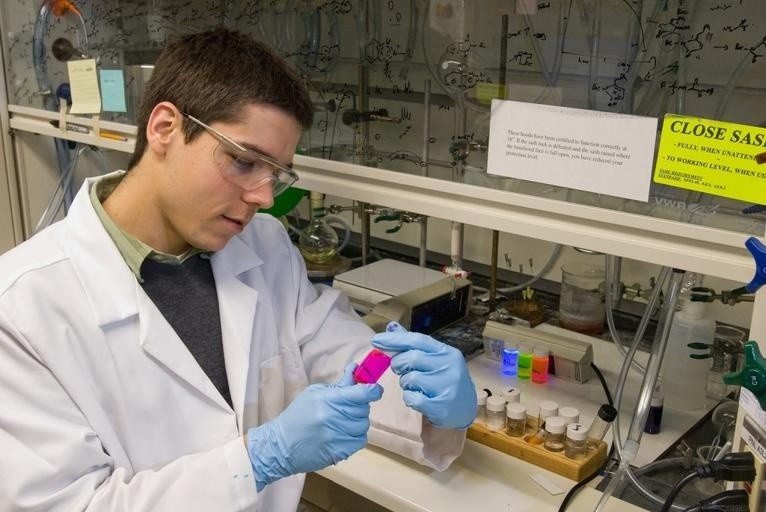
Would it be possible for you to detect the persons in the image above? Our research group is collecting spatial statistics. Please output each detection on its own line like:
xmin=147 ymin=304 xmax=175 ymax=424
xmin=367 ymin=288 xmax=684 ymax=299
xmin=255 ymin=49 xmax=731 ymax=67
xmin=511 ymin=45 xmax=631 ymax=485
xmin=0 ymin=29 xmax=479 ymax=511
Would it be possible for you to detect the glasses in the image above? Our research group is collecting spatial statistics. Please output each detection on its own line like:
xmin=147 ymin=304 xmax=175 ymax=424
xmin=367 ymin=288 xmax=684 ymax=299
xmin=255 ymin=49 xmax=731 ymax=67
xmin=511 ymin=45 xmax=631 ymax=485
xmin=181 ymin=110 xmax=299 ymax=197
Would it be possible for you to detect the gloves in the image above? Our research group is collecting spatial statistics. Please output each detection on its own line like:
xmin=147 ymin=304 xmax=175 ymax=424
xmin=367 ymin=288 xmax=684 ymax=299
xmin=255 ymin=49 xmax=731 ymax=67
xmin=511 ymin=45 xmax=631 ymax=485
xmin=247 ymin=363 xmax=383 ymax=490
xmin=372 ymin=321 xmax=477 ymax=431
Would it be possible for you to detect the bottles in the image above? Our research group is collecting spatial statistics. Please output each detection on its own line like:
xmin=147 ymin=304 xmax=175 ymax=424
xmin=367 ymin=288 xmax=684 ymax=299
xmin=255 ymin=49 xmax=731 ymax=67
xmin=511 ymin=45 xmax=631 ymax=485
xmin=502 ymin=339 xmax=550 ymax=383
xmin=477 ymin=386 xmax=618 ymax=460
xmin=712 ymin=326 xmax=744 ymax=399
xmin=298 ymin=191 xmax=339 ymax=263
xmin=663 ymin=301 xmax=716 ymax=407
xmin=354 ymin=345 xmax=395 ymax=384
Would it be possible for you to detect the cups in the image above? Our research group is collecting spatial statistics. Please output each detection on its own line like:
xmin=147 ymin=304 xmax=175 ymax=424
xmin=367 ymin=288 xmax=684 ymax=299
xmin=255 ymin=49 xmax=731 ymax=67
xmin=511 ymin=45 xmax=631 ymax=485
xmin=560 ymin=263 xmax=610 ymax=335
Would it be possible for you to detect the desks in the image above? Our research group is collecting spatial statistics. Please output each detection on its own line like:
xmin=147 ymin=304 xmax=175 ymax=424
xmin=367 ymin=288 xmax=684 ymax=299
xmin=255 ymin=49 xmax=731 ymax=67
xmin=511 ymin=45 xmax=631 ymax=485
xmin=309 ymin=323 xmax=740 ymax=512
